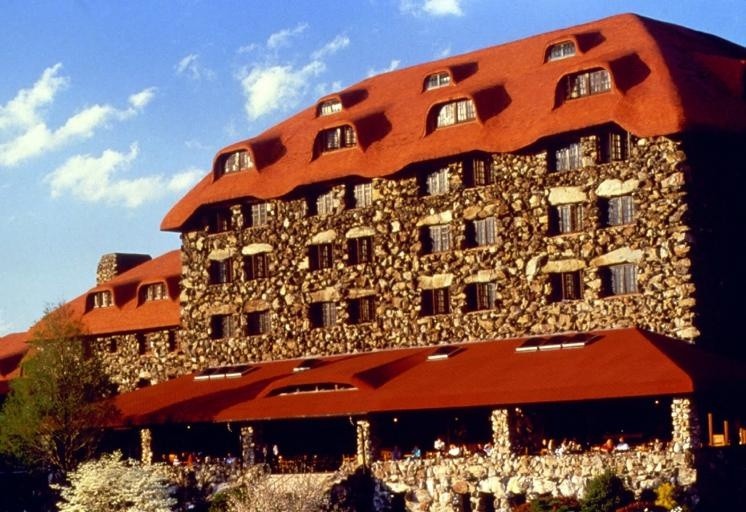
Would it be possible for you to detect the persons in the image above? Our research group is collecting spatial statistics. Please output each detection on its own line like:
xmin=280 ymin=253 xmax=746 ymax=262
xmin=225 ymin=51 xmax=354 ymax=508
xmin=404 ymin=437 xmax=630 ymax=459
xmin=162 ymin=447 xmax=278 ymax=469
